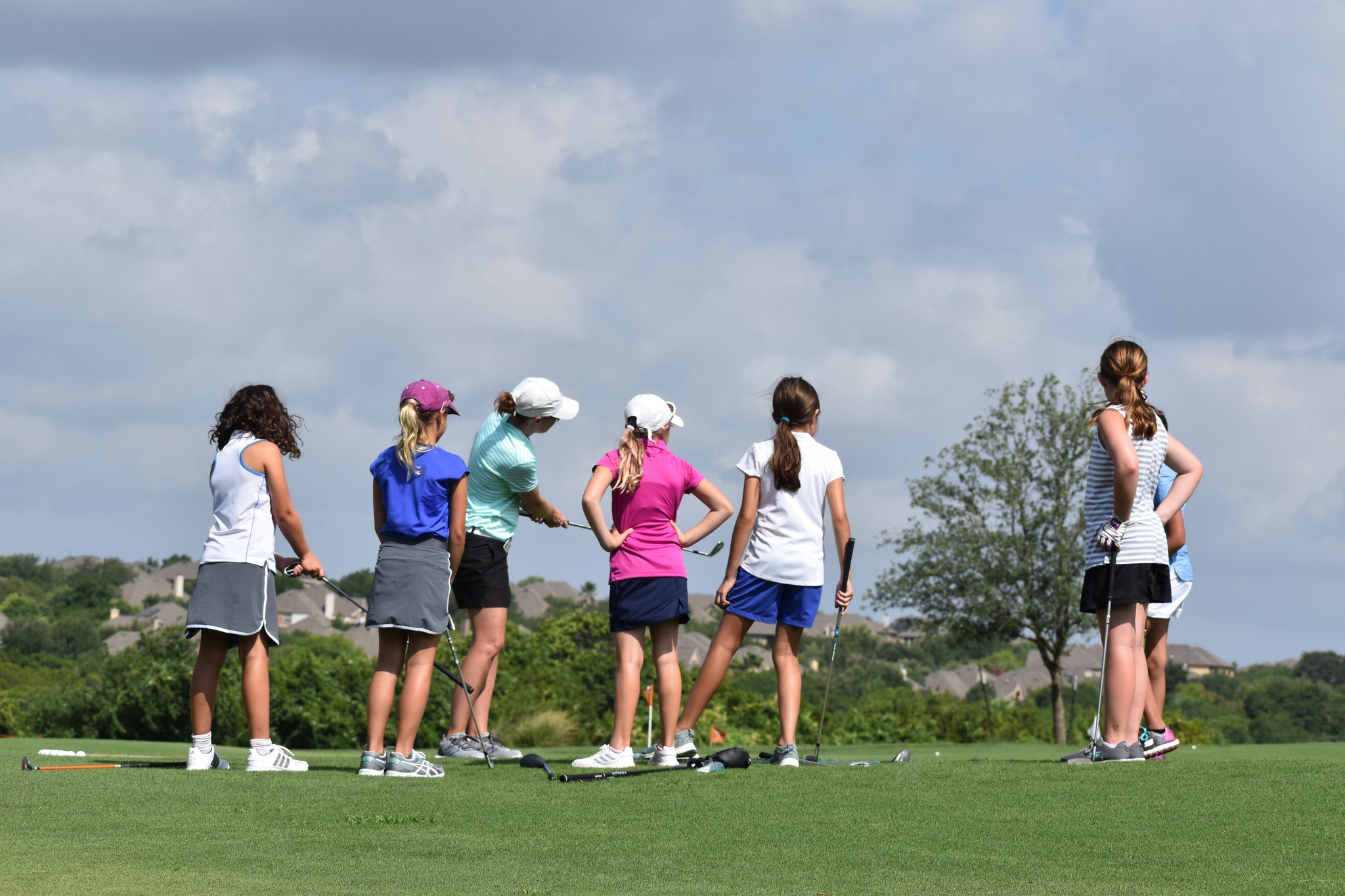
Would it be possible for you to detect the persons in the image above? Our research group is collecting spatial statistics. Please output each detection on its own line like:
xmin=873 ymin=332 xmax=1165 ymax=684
xmin=641 ymin=377 xmax=854 ymax=765
xmin=571 ymin=393 xmax=734 ymax=769
xmin=359 ymin=379 xmax=470 ymax=778
xmin=1060 ymin=340 xmax=1203 ymax=763
xmin=184 ymin=385 xmax=326 ymax=771
xmin=1138 ymin=462 xmax=1194 ymax=759
xmin=435 ymin=377 xmax=580 ymax=760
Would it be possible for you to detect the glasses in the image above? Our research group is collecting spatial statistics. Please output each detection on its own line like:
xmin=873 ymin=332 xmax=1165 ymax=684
xmin=549 ymin=417 xmax=560 ymax=422
xmin=440 ymin=390 xmax=455 ymax=413
xmin=662 ymin=402 xmax=676 ymax=429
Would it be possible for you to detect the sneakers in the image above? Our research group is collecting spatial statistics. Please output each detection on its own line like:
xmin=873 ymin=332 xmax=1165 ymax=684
xmin=770 ymin=743 xmax=799 ymax=768
xmin=648 ymin=745 xmax=680 ymax=767
xmin=1060 ymin=736 xmax=1130 ymax=763
xmin=467 ymin=731 xmax=523 ymax=759
xmin=386 ymin=746 xmax=445 ymax=778
xmin=245 ymin=744 xmax=309 ymax=772
xmin=1139 ymin=724 xmax=1180 ymax=760
xmin=358 ymin=744 xmax=396 ymax=777
xmin=187 ymin=743 xmax=230 ymax=770
xmin=572 ymin=744 xmax=635 ymax=768
xmin=1127 ymin=740 xmax=1145 ymax=761
xmin=435 ymin=732 xmax=485 ymax=758
xmin=640 ymin=729 xmax=697 ymax=759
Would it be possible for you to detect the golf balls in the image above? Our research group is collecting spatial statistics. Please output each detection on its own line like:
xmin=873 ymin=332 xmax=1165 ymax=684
xmin=935 ymin=752 xmax=940 ymax=756
xmin=37 ymin=749 xmax=86 ymax=757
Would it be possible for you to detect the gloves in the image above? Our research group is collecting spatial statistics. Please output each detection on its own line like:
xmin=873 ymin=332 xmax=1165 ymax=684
xmin=1096 ymin=513 xmax=1130 ymax=553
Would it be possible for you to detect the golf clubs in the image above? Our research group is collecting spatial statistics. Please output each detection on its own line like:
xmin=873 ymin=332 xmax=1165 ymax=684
xmin=22 ymin=756 xmax=187 ymax=771
xmin=283 ymin=560 xmax=474 ymax=694
xmin=751 ymin=749 xmax=911 ymax=767
xmin=559 ymin=756 xmax=706 ymax=783
xmin=445 ymin=626 xmax=496 ymax=770
xmin=804 ymin=536 xmax=856 ymax=762
xmin=518 ymin=509 xmax=725 ymax=557
xmin=1066 ymin=544 xmax=1117 ymax=766
xmin=520 ymin=753 xmax=556 ymax=781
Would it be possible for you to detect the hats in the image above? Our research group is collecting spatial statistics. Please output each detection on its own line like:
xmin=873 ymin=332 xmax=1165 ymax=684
xmin=400 ymin=380 xmax=461 ymax=417
xmin=624 ymin=394 xmax=684 ymax=445
xmin=509 ymin=377 xmax=579 ymax=420
